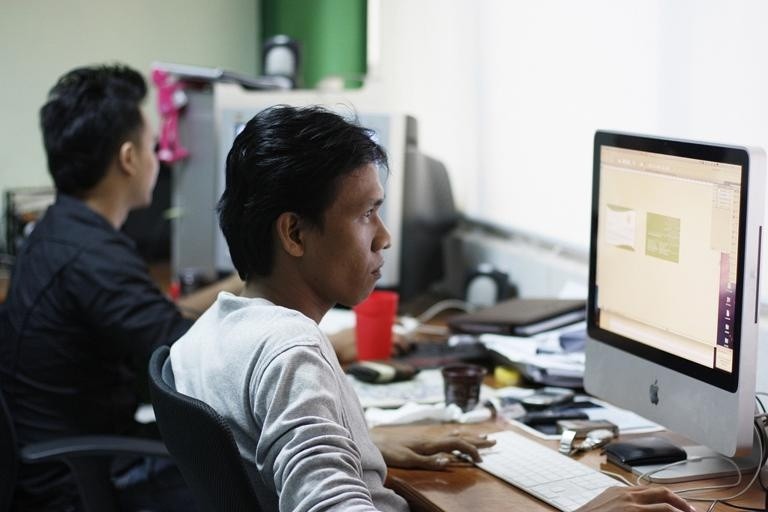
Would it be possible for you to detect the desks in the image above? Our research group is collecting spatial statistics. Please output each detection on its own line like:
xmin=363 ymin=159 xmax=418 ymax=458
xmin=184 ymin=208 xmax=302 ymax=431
xmin=368 ymin=307 xmax=768 ymax=510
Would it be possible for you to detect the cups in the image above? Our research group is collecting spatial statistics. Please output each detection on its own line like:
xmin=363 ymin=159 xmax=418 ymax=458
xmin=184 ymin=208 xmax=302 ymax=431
xmin=353 ymin=290 xmax=399 ymax=360
xmin=442 ymin=365 xmax=488 ymax=413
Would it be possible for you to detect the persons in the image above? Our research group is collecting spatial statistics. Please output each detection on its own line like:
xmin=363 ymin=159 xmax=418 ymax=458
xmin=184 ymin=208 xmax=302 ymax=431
xmin=1 ymin=66 xmax=407 ymax=512
xmin=166 ymin=104 xmax=697 ymax=512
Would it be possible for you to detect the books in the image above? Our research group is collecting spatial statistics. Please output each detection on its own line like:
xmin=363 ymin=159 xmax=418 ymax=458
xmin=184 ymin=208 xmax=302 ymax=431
xmin=444 ymin=297 xmax=587 ymax=336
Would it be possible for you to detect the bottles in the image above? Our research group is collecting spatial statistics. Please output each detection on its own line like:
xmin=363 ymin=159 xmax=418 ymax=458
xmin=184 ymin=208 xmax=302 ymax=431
xmin=265 ymin=35 xmax=297 ymax=79
xmin=465 ymin=263 xmax=498 ymax=306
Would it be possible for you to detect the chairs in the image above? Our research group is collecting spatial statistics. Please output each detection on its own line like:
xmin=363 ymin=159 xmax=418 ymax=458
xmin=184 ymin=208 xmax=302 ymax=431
xmin=0 ymin=387 xmax=167 ymax=511
xmin=146 ymin=346 xmax=260 ymax=511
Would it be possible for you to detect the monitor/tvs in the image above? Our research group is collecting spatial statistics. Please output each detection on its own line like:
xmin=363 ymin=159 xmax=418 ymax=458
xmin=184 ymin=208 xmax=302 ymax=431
xmin=582 ymin=126 xmax=768 ymax=484
xmin=214 ymin=88 xmax=409 ymax=290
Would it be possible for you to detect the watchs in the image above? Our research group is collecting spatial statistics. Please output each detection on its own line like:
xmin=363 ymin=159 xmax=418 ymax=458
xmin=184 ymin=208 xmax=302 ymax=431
xmin=559 ymin=425 xmax=614 ymax=458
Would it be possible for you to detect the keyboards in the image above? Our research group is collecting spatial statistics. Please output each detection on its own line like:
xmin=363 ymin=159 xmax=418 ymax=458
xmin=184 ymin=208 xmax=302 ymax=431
xmin=451 ymin=429 xmax=630 ymax=512
xmin=318 ymin=307 xmax=357 ymax=334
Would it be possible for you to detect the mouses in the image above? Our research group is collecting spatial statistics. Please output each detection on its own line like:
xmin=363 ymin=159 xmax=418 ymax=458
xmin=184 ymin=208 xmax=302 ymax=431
xmin=392 ymin=316 xmax=419 ymax=335
